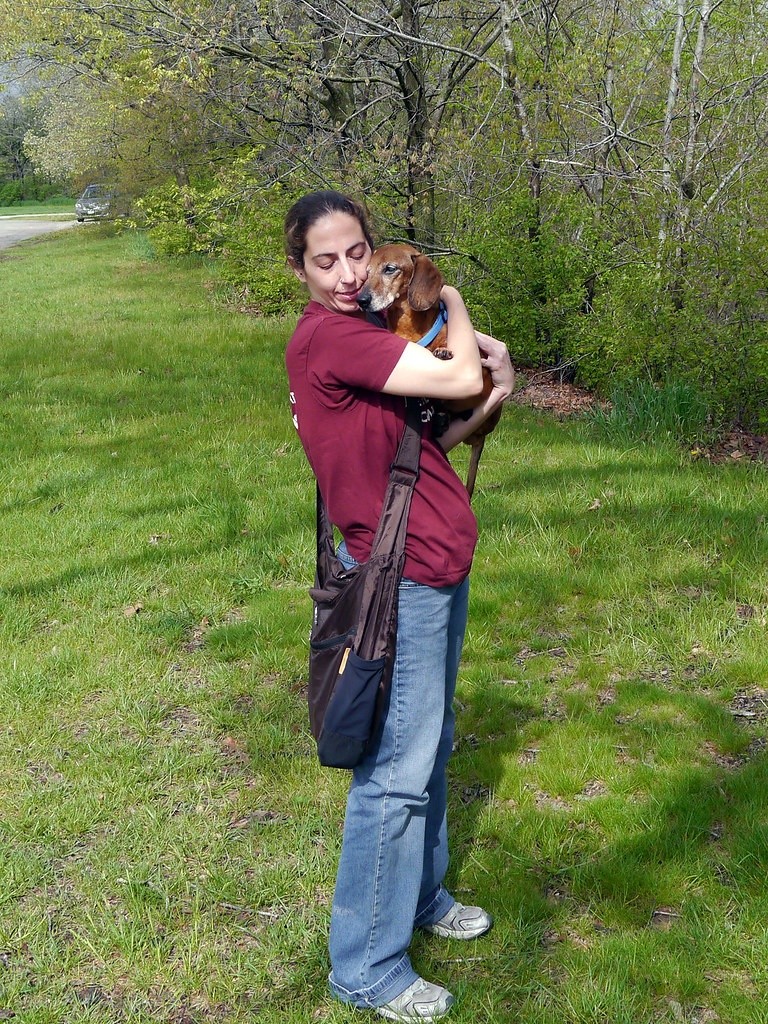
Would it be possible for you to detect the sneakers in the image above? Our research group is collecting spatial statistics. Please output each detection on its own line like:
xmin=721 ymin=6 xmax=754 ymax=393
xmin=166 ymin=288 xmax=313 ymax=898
xmin=371 ymin=977 xmax=456 ymax=1024
xmin=422 ymin=902 xmax=492 ymax=940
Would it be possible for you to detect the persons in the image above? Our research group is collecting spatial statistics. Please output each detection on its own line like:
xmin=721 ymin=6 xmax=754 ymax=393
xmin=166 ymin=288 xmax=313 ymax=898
xmin=285 ymin=189 xmax=514 ymax=1024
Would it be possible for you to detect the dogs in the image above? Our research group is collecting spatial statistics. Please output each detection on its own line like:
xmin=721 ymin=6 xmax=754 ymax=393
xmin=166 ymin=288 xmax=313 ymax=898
xmin=357 ymin=242 xmax=503 ymax=504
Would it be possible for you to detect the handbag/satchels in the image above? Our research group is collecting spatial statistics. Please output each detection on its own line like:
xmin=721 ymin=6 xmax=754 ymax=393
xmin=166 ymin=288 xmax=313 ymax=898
xmin=308 ymin=530 xmax=406 ymax=769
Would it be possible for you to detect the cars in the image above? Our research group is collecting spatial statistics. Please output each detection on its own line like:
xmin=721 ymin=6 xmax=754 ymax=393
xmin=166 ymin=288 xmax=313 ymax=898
xmin=76 ymin=184 xmax=134 ymax=223
